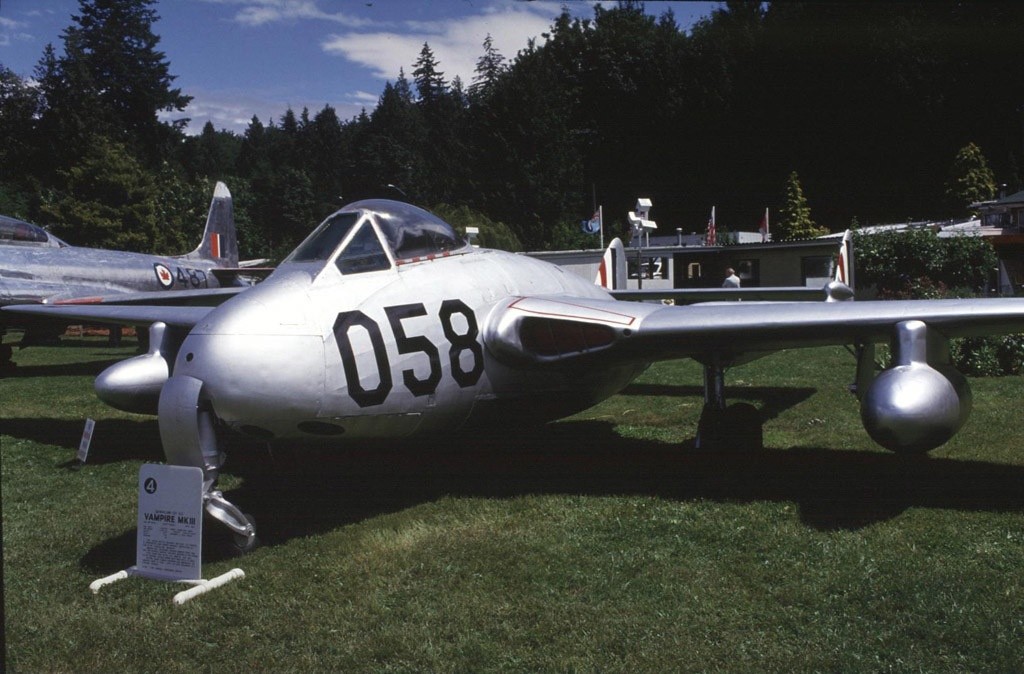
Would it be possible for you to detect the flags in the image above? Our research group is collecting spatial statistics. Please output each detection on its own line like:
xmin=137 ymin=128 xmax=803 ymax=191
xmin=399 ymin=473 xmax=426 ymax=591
xmin=758 ymin=213 xmax=768 ymax=242
xmin=581 ymin=209 xmax=601 ymax=235
xmin=705 ymin=217 xmax=716 ymax=246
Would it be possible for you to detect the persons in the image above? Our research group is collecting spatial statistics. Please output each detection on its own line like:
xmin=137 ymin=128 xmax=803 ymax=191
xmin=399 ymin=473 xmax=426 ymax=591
xmin=722 ymin=267 xmax=741 ymax=301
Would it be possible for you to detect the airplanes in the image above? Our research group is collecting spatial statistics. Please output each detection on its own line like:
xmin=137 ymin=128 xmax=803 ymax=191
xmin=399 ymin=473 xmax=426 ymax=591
xmin=1 ymin=200 xmax=1022 ymax=559
xmin=1 ymin=179 xmax=276 ymax=353
